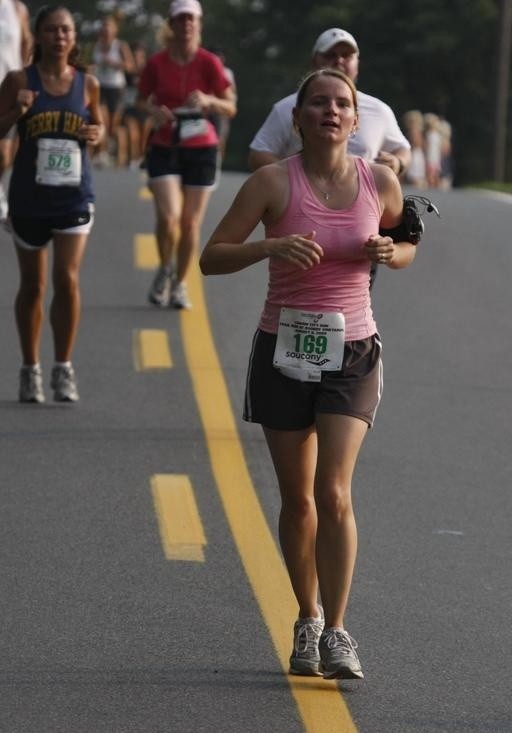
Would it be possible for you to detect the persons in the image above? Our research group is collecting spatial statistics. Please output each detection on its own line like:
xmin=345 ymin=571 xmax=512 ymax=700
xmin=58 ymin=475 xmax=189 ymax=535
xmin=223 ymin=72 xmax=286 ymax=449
xmin=403 ymin=108 xmax=456 ymax=190
xmin=132 ymin=0 xmax=239 ymax=310
xmin=198 ymin=66 xmax=421 ymax=678
xmin=0 ymin=0 xmax=34 ymax=223
xmin=211 ymin=48 xmax=238 ymax=154
xmin=249 ymin=25 xmax=415 ymax=293
xmin=91 ymin=13 xmax=127 ymax=172
xmin=124 ymin=41 xmax=153 ymax=162
xmin=0 ymin=0 xmax=107 ymax=406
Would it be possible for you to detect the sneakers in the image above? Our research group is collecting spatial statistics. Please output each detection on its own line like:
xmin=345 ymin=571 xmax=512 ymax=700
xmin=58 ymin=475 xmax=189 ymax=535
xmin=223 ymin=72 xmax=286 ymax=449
xmin=149 ymin=267 xmax=173 ymax=307
xmin=170 ymin=282 xmax=190 ymax=309
xmin=318 ymin=626 xmax=364 ymax=678
xmin=49 ymin=365 xmax=80 ymax=402
xmin=19 ymin=366 xmax=46 ymax=404
xmin=289 ymin=603 xmax=326 ymax=676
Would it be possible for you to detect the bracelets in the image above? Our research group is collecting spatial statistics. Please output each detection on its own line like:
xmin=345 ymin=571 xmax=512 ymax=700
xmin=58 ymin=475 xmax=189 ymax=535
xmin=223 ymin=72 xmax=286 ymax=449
xmin=398 ymin=157 xmax=405 ymax=177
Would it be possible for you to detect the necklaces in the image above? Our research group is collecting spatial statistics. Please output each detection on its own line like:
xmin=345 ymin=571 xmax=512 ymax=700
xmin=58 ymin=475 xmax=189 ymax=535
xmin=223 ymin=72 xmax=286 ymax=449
xmin=306 ymin=168 xmax=343 ymax=202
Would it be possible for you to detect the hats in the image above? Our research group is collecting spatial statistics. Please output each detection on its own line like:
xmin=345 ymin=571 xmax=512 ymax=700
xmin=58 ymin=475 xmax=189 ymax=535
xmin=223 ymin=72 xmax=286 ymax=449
xmin=309 ymin=27 xmax=360 ymax=62
xmin=168 ymin=0 xmax=202 ymax=20
